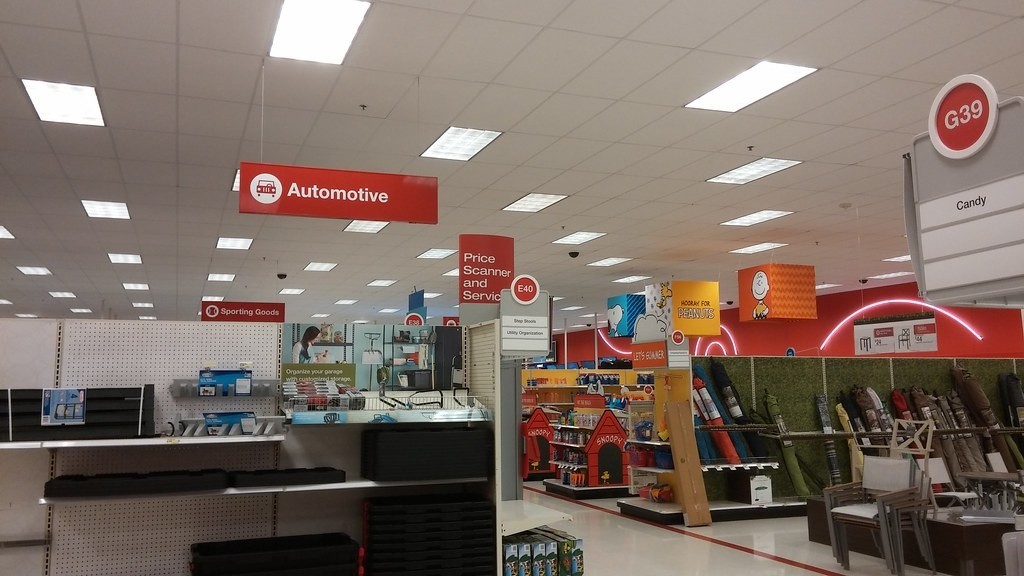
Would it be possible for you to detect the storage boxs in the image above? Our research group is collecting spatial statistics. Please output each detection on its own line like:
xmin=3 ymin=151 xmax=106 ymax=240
xmin=0 ymin=331 xmax=675 ymax=576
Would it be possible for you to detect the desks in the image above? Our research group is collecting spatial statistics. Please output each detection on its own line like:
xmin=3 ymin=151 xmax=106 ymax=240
xmin=955 ymin=471 xmax=1021 ymax=510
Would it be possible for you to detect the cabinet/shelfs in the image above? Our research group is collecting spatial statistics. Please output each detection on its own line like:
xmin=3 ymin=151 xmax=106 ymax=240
xmin=514 ymin=358 xmax=1024 ymax=526
xmin=0 ymin=317 xmax=502 ymax=575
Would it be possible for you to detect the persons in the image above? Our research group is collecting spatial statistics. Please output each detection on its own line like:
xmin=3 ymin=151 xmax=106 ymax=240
xmin=293 ymin=326 xmax=320 ymax=364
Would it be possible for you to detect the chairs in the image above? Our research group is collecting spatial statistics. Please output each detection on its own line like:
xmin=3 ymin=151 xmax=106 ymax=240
xmin=823 ymin=419 xmax=986 ymax=576
xmin=986 ymin=452 xmax=1024 ymax=515
